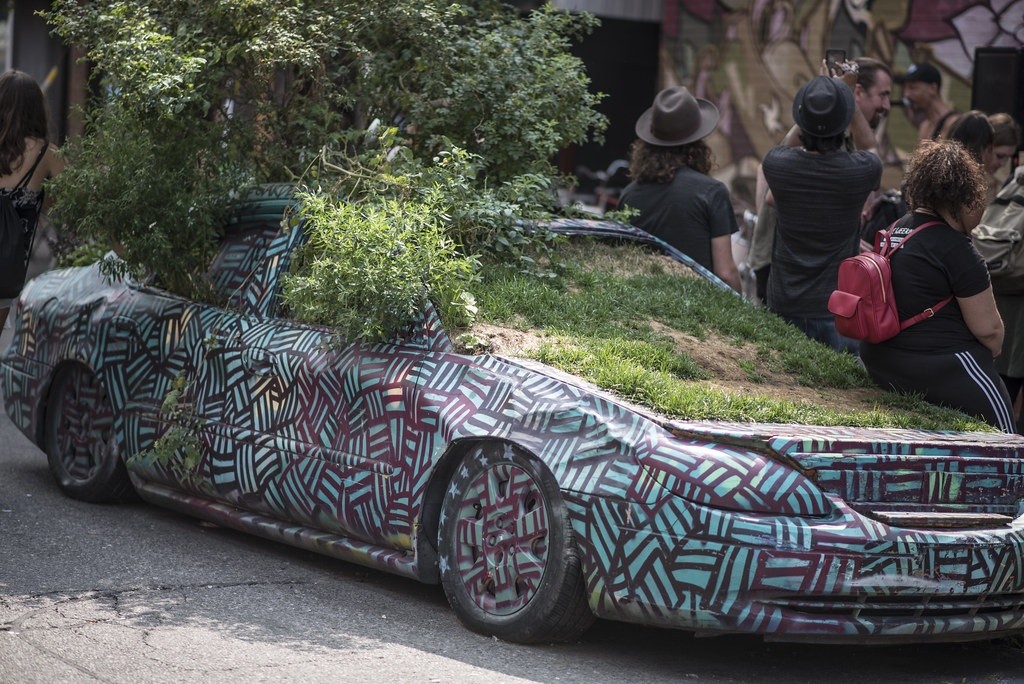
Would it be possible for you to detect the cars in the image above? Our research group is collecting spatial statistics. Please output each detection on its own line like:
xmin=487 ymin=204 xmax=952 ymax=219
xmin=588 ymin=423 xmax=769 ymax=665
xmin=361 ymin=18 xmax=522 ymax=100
xmin=2 ymin=182 xmax=1024 ymax=654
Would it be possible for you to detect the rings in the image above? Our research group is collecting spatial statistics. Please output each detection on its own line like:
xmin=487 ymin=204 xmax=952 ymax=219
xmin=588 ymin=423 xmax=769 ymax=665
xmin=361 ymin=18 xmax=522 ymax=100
xmin=833 ymin=75 xmax=838 ymax=77
xmin=842 ymin=62 xmax=859 ymax=74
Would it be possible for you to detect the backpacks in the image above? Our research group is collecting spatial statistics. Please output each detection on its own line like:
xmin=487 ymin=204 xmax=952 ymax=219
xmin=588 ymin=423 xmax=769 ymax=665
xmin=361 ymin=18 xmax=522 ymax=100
xmin=827 ymin=216 xmax=952 ymax=343
xmin=0 ymin=139 xmax=49 ymax=296
xmin=969 ymin=166 xmax=1024 ymax=278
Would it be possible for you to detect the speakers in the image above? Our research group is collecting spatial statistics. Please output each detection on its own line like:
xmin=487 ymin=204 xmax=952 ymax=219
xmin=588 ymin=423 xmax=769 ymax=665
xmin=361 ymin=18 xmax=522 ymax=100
xmin=971 ymin=47 xmax=1024 ymax=151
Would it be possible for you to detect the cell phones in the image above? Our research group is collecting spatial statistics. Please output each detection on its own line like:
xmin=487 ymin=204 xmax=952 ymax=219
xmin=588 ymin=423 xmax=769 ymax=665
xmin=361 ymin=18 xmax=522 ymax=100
xmin=826 ymin=49 xmax=846 ymax=77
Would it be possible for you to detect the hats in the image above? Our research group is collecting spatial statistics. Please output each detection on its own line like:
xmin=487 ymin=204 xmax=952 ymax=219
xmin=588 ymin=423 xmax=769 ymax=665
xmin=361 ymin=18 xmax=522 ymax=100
xmin=792 ymin=76 xmax=855 ymax=137
xmin=636 ymin=84 xmax=715 ymax=146
xmin=893 ymin=61 xmax=941 ymax=84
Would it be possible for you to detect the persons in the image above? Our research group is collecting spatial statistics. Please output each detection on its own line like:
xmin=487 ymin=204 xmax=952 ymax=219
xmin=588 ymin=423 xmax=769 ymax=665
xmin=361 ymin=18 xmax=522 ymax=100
xmin=0 ymin=68 xmax=76 ymax=332
xmin=762 ymin=58 xmax=885 ymax=351
xmin=841 ymin=58 xmax=893 ymax=154
xmin=860 ymin=141 xmax=1017 ymax=434
xmin=746 ymin=164 xmax=777 ymax=303
xmin=892 ymin=62 xmax=957 ymax=144
xmin=941 ymin=112 xmax=1024 ymax=244
xmin=616 ymin=84 xmax=742 ymax=295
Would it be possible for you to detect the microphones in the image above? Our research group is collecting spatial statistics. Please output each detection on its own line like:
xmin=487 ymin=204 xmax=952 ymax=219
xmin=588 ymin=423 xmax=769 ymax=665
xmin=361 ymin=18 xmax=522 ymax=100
xmin=890 ymin=99 xmax=912 ymax=107
